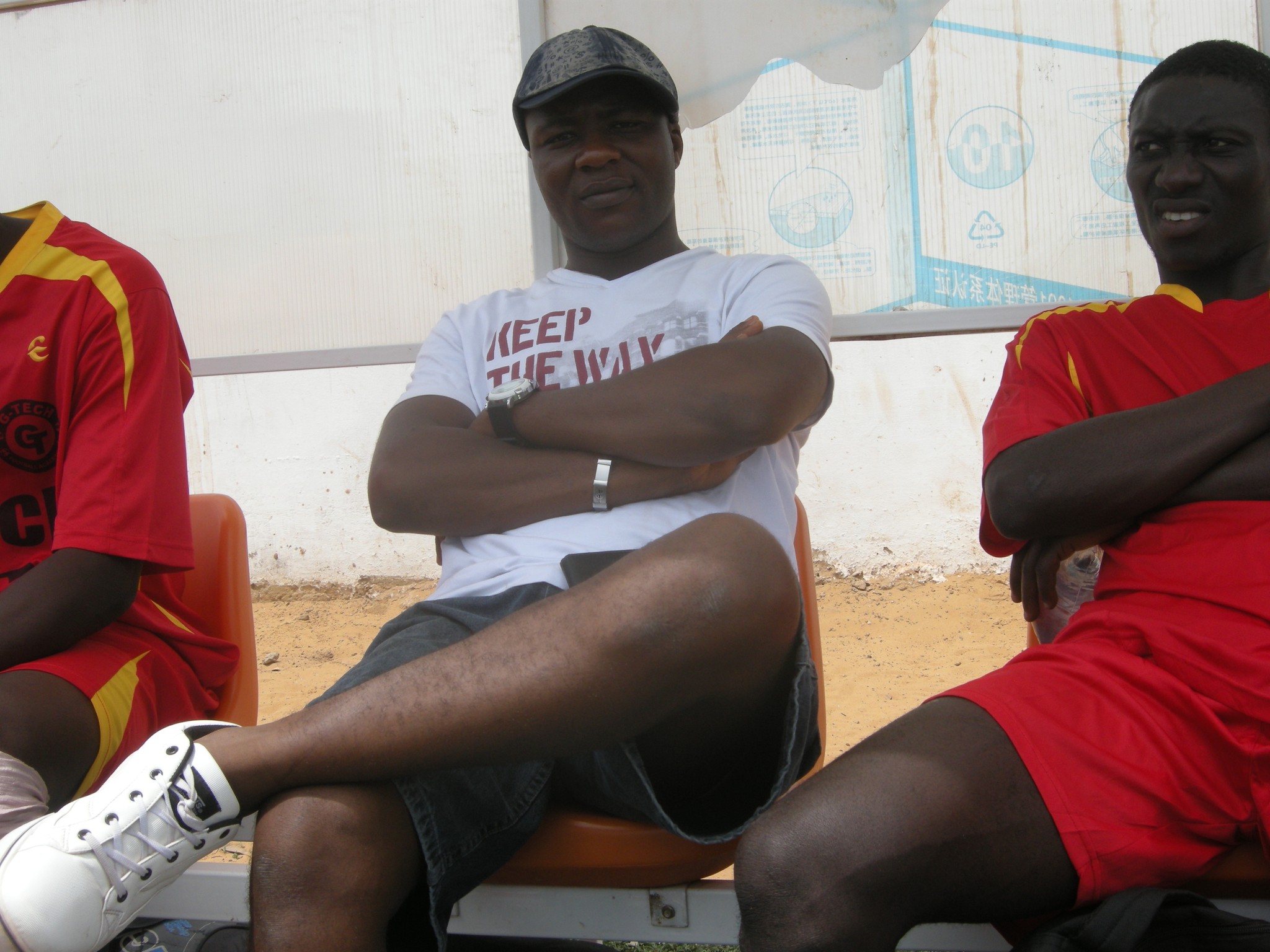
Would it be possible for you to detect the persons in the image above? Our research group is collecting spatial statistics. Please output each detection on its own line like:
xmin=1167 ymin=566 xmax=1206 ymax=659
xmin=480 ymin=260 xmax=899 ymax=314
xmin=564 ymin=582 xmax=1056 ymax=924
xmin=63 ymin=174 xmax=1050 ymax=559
xmin=732 ymin=41 xmax=1270 ymax=952
xmin=0 ymin=23 xmax=835 ymax=952
xmin=1 ymin=202 xmax=240 ymax=844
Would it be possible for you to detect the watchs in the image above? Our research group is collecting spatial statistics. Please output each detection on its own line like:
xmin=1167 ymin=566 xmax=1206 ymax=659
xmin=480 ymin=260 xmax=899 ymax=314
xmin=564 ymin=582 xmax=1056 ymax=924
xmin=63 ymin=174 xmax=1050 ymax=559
xmin=486 ymin=377 xmax=542 ymax=448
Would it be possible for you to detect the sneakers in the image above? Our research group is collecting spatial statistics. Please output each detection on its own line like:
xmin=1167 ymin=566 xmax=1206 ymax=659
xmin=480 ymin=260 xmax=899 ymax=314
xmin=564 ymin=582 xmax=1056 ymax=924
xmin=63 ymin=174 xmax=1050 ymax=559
xmin=0 ymin=720 xmax=264 ymax=952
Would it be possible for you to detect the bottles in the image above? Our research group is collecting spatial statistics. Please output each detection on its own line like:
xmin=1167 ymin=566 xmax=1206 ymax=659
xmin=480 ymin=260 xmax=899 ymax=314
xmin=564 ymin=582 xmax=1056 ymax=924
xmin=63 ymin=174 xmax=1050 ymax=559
xmin=1026 ymin=545 xmax=1104 ymax=648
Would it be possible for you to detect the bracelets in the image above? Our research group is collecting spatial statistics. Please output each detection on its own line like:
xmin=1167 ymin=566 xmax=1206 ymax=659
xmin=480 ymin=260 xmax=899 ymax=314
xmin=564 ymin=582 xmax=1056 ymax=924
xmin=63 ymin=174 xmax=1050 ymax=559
xmin=593 ymin=455 xmax=614 ymax=512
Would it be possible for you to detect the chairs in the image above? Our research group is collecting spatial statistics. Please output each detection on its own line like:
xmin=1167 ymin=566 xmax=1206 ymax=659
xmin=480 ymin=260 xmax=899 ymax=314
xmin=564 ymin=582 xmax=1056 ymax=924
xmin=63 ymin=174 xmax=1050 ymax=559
xmin=435 ymin=496 xmax=823 ymax=883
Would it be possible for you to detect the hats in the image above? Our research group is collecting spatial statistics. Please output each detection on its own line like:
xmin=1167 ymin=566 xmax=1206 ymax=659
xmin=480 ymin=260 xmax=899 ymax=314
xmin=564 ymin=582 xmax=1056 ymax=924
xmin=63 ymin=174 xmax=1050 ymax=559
xmin=512 ymin=24 xmax=679 ymax=151
